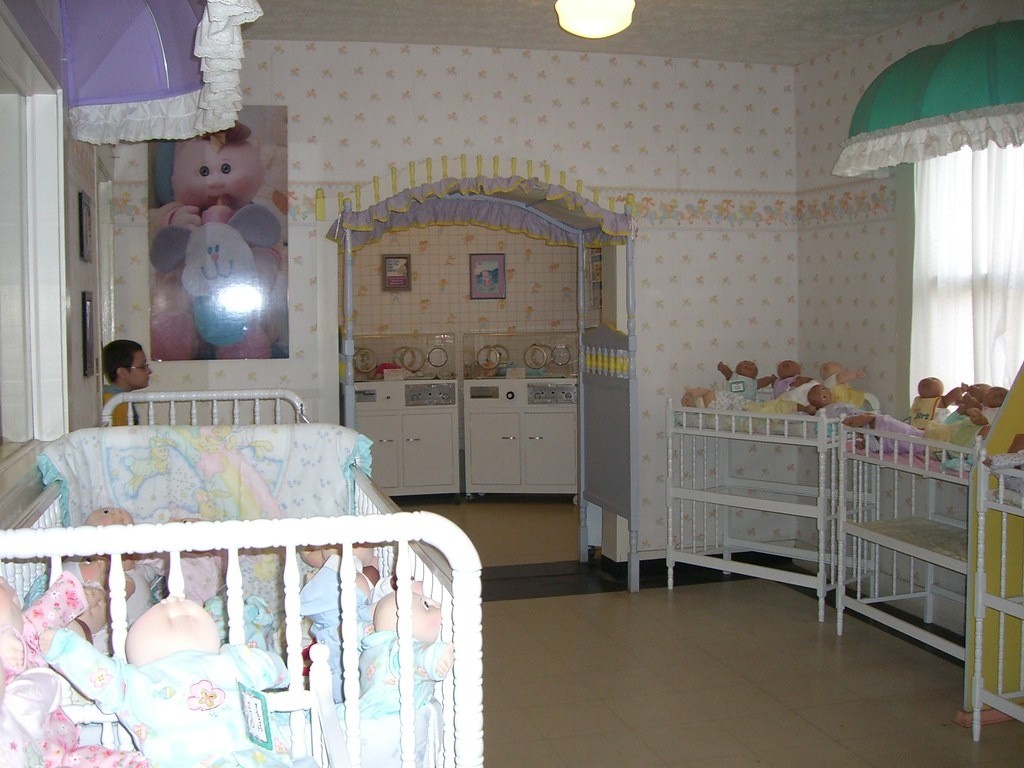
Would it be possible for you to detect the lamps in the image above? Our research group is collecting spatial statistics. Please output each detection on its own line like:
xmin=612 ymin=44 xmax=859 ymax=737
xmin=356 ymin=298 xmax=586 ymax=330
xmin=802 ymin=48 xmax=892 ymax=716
xmin=555 ymin=0 xmax=636 ymax=40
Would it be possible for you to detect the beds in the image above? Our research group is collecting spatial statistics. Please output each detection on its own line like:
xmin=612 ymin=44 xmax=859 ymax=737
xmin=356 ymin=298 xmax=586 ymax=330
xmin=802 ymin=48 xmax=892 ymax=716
xmin=0 ymin=391 xmax=484 ymax=768
xmin=835 ymin=411 xmax=982 ymax=709
xmin=970 ymin=447 xmax=1024 ymax=743
xmin=667 ymin=397 xmax=880 ymax=623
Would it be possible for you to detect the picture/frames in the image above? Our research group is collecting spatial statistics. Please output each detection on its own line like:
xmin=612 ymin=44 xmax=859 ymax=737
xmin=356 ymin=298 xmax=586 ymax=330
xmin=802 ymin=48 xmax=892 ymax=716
xmin=81 ymin=190 xmax=92 ymax=263
xmin=83 ymin=291 xmax=95 ymax=378
xmin=469 ymin=253 xmax=506 ymax=300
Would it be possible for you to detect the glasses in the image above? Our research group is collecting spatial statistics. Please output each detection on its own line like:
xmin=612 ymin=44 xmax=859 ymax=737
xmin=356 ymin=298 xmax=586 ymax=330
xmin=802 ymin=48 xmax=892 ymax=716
xmin=130 ymin=364 xmax=148 ymax=371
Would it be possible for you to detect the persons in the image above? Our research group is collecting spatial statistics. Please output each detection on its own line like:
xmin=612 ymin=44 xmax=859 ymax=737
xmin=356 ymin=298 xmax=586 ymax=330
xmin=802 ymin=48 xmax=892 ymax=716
xmin=682 ymin=359 xmax=1010 ymax=465
xmin=0 ymin=505 xmax=453 ymax=768
xmin=102 ymin=339 xmax=152 ymax=426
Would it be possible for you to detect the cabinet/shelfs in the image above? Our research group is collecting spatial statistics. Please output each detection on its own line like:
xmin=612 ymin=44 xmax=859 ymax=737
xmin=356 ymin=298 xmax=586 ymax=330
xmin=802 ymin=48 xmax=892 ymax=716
xmin=464 ymin=379 xmax=579 ymax=505
xmin=353 ymin=380 xmax=460 ymax=496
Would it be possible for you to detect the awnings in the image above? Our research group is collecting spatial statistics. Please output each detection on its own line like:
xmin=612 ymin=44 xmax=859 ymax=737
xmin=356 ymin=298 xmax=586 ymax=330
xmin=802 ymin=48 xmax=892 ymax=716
xmin=829 ymin=18 xmax=1024 ymax=178
